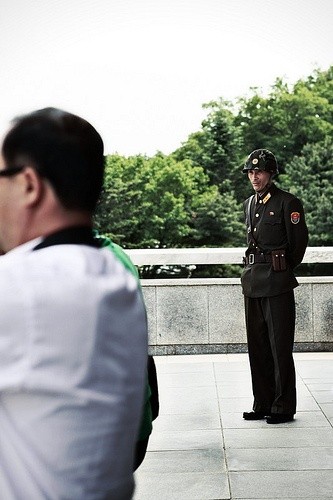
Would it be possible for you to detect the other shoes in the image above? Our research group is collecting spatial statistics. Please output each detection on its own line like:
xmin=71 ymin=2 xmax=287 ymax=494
xmin=243 ymin=411 xmax=263 ymax=420
xmin=264 ymin=413 xmax=293 ymax=422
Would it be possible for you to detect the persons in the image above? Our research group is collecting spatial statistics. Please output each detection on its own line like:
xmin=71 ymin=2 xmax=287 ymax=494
xmin=0 ymin=107 xmax=160 ymax=500
xmin=240 ymin=149 xmax=309 ymax=424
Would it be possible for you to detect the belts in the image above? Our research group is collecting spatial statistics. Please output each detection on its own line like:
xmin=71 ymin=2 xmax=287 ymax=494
xmin=243 ymin=254 xmax=273 ymax=265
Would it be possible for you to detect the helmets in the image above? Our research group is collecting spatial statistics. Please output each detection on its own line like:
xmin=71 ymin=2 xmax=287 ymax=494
xmin=242 ymin=148 xmax=280 ymax=175
